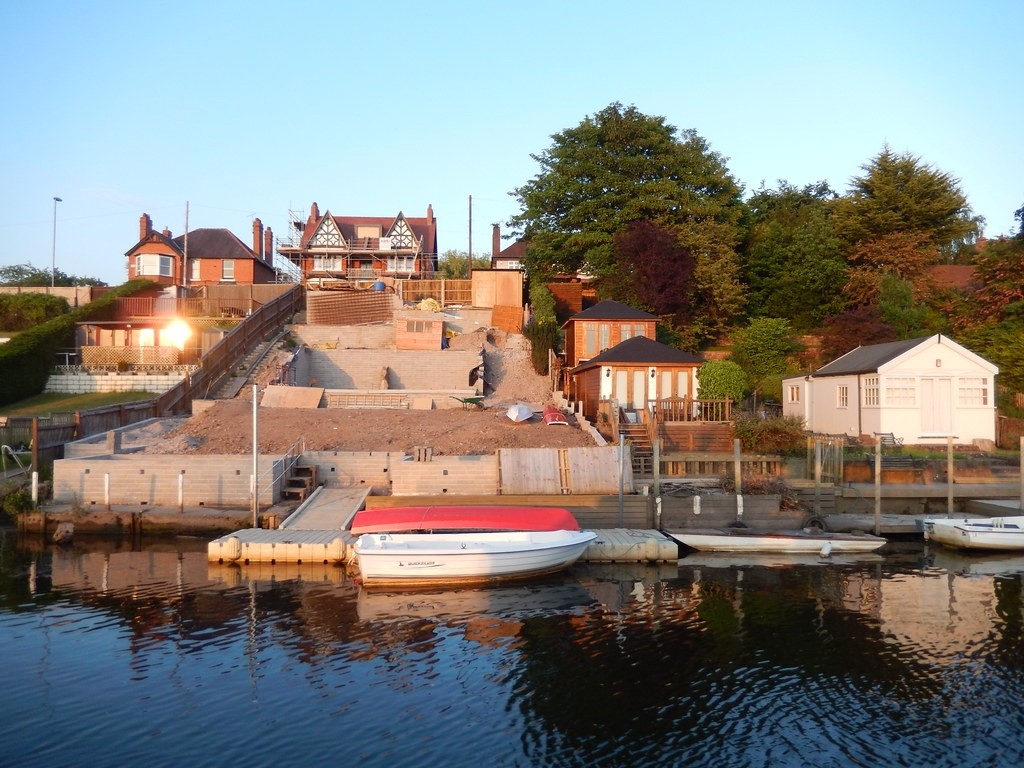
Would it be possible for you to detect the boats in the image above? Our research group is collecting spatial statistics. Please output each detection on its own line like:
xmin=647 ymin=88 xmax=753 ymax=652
xmin=925 ymin=516 xmax=1024 ymax=550
xmin=352 ymin=533 xmax=600 ymax=589
xmin=664 ymin=530 xmax=888 ymax=553
xmin=350 ymin=507 xmax=581 ymax=536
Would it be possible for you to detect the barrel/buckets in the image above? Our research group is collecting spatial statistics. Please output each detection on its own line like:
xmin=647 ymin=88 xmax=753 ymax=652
xmin=374 ymin=281 xmax=385 ymax=292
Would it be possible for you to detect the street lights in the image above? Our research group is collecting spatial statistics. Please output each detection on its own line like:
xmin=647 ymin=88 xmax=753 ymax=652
xmin=52 ymin=196 xmax=61 ymax=286
